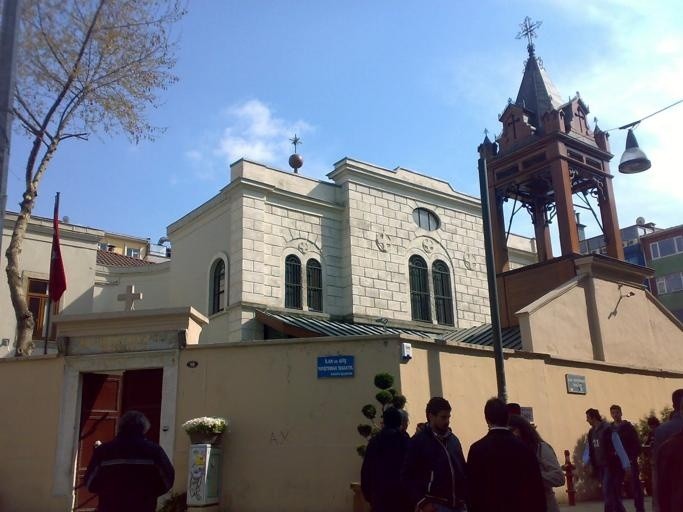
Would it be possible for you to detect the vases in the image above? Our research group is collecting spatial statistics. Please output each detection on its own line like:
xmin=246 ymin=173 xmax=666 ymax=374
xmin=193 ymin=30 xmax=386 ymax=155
xmin=185 ymin=444 xmax=221 ymax=507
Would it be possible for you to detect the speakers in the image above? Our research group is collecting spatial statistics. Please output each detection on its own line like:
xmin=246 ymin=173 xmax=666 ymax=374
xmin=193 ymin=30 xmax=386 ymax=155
xmin=402 ymin=342 xmax=413 ymax=360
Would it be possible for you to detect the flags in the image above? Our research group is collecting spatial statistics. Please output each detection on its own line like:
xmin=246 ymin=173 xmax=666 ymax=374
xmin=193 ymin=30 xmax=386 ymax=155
xmin=46 ymin=190 xmax=67 ymax=304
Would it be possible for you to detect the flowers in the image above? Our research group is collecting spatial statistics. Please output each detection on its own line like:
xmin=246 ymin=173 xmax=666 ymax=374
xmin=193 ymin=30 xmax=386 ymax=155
xmin=180 ymin=416 xmax=227 ymax=445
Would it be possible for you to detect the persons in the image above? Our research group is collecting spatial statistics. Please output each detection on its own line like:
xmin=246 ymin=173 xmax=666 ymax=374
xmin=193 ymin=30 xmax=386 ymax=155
xmin=80 ymin=410 xmax=174 ymax=512
xmin=508 ymin=415 xmax=565 ymax=512
xmin=605 ymin=404 xmax=646 ymax=512
xmin=641 ymin=412 xmax=661 ymax=467
xmin=650 ymin=388 xmax=683 ymax=512
xmin=581 ymin=408 xmax=632 ymax=512
xmin=359 ymin=406 xmax=424 ymax=512
xmin=408 ymin=394 xmax=468 ymax=512
xmin=467 ymin=395 xmax=547 ymax=512
